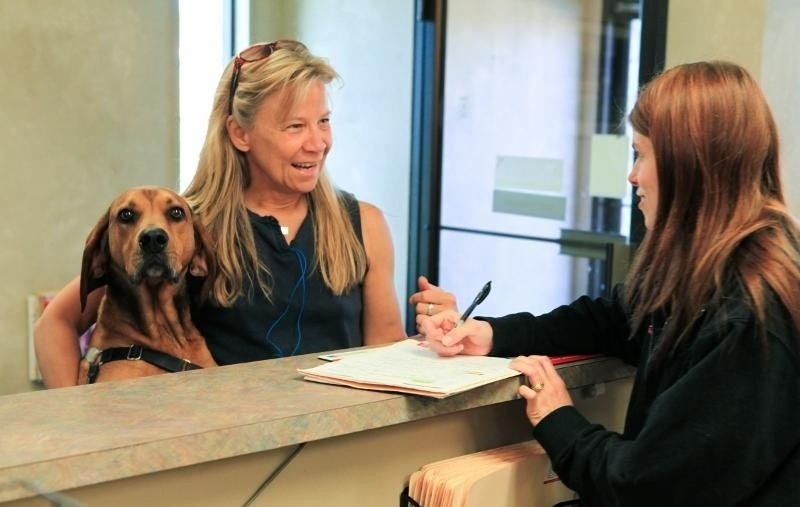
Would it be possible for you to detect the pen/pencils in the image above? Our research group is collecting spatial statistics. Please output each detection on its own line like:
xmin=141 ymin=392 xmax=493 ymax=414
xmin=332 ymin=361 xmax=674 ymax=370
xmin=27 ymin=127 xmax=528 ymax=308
xmin=454 ymin=281 xmax=493 ymax=331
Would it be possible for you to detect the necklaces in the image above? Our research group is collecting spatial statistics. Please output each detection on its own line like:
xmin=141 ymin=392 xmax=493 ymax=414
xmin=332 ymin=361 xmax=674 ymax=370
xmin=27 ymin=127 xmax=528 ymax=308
xmin=281 ymin=226 xmax=290 ymax=235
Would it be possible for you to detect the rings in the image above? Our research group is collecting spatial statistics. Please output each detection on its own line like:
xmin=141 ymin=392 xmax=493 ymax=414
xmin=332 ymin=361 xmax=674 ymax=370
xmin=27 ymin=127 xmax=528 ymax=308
xmin=530 ymin=380 xmax=545 ymax=391
xmin=427 ymin=303 xmax=433 ymax=315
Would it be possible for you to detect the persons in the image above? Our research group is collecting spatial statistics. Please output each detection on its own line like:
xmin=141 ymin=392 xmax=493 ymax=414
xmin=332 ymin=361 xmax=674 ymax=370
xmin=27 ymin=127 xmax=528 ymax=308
xmin=30 ymin=35 xmax=461 ymax=393
xmin=423 ymin=61 xmax=800 ymax=507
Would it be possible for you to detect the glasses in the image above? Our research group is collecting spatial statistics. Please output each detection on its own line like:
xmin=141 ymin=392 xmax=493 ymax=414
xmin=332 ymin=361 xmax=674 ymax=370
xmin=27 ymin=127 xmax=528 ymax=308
xmin=228 ymin=39 xmax=309 ymax=115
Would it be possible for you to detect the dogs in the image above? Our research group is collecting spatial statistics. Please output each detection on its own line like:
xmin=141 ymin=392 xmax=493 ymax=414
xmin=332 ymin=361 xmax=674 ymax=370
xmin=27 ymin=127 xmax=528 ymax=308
xmin=75 ymin=184 xmax=219 ymax=388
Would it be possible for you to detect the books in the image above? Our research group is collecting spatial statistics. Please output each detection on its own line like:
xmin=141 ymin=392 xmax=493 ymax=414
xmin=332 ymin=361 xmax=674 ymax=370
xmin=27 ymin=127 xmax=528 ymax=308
xmin=293 ymin=337 xmax=524 ymax=402
